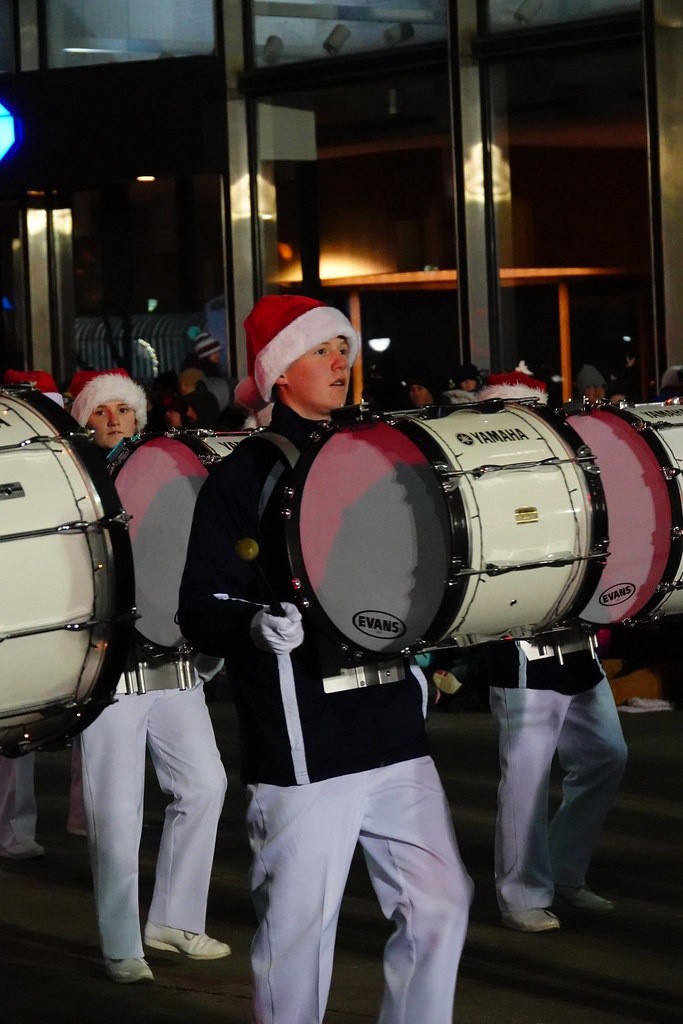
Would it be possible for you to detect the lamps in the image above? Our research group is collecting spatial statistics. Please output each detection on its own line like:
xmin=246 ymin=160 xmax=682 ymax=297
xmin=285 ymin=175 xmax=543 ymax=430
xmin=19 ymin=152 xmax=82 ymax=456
xmin=383 ymin=23 xmax=413 ymax=42
xmin=514 ymin=0 xmax=541 ymax=27
xmin=263 ymin=35 xmax=282 ymax=63
xmin=323 ymin=25 xmax=350 ymax=53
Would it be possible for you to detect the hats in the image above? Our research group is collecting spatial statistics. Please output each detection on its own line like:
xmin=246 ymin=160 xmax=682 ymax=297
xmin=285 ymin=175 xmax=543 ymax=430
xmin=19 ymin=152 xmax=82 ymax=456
xmin=404 ymin=370 xmax=436 ymax=396
xmin=232 ymin=294 xmax=359 ymax=412
xmin=3 ymin=367 xmax=65 ymax=411
xmin=67 ymin=368 xmax=149 ymax=434
xmin=474 ymin=370 xmax=550 ymax=408
xmin=186 ymin=325 xmax=224 ymax=359
xmin=659 ymin=365 xmax=683 ymax=389
xmin=575 ymin=363 xmax=609 ymax=398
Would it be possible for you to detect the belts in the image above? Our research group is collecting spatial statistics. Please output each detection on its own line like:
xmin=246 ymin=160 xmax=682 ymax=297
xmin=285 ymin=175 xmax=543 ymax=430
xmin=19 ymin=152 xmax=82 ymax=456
xmin=321 ymin=659 xmax=409 ymax=694
xmin=515 ymin=634 xmax=601 ymax=660
xmin=111 ymin=658 xmax=199 ymax=697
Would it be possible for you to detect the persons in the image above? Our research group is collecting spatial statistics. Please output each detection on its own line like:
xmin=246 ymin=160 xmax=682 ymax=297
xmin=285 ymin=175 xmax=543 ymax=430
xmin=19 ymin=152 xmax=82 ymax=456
xmin=0 ymin=370 xmax=91 ymax=864
xmin=179 ymin=295 xmax=475 ymax=1024
xmin=476 ymin=371 xmax=628 ymax=932
xmin=68 ymin=368 xmax=232 ymax=985
xmin=56 ymin=337 xmax=683 ymax=433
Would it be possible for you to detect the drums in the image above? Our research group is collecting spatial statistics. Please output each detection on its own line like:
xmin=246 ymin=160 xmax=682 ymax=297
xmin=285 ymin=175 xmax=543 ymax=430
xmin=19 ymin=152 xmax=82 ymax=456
xmin=280 ymin=397 xmax=613 ymax=662
xmin=0 ymin=379 xmax=137 ymax=756
xmin=102 ymin=426 xmax=269 ymax=669
xmin=554 ymin=397 xmax=683 ymax=636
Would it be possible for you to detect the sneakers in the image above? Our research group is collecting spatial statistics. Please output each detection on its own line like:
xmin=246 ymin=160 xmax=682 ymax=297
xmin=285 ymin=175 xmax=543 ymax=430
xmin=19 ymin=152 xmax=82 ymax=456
xmin=499 ymin=908 xmax=563 ymax=934
xmin=554 ymin=883 xmax=616 ymax=915
xmin=66 ymin=824 xmax=89 ymax=837
xmin=143 ymin=918 xmax=231 ymax=961
xmin=104 ymin=956 xmax=156 ymax=985
xmin=0 ymin=839 xmax=45 ymax=861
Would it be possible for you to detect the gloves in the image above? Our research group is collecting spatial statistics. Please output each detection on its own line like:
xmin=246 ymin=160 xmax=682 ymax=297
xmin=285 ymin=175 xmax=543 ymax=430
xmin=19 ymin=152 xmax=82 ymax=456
xmin=250 ymin=601 xmax=305 ymax=656
